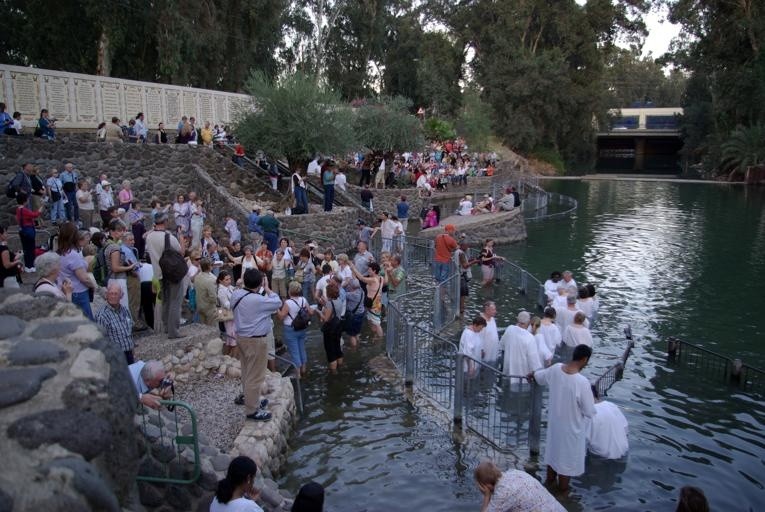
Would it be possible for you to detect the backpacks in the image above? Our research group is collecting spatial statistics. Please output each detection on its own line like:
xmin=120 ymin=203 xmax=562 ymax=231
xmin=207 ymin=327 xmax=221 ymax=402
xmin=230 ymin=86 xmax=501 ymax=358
xmin=159 ymin=232 xmax=189 ymax=282
xmin=344 ymin=310 xmax=353 ymax=330
xmin=289 ymin=297 xmax=311 ymax=330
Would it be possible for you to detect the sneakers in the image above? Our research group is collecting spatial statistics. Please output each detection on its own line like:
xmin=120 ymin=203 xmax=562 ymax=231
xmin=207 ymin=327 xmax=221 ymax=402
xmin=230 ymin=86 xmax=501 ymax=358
xmin=259 ymin=398 xmax=269 ymax=410
xmin=245 ymin=410 xmax=272 ymax=422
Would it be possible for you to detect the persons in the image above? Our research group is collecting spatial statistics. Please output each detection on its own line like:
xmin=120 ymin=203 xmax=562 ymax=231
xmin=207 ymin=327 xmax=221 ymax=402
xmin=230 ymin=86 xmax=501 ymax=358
xmin=586 ymin=383 xmax=629 ymax=462
xmin=528 ymin=345 xmax=597 ymax=494
xmin=676 ymin=485 xmax=709 ymax=512
xmin=475 ymin=462 xmax=567 ymax=512
xmin=210 ymin=457 xmax=264 ymax=512
xmin=288 ymin=480 xmax=325 ymax=512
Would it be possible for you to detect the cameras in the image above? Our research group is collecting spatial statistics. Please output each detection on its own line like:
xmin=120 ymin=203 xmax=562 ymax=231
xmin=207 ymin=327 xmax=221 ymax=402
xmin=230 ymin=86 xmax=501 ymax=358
xmin=160 ymin=376 xmax=174 ymax=389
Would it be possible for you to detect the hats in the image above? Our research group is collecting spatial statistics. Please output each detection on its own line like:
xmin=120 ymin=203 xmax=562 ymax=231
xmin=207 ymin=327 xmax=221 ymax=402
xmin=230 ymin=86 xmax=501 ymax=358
xmin=101 ymin=180 xmax=111 ymax=187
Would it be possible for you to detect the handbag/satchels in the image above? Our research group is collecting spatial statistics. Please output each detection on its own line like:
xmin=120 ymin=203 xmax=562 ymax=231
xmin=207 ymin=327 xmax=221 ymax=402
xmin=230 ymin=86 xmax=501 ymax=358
xmin=363 ymin=295 xmax=373 ymax=308
xmin=320 ymin=300 xmax=341 ymax=336
xmin=51 ymin=189 xmax=62 ymax=202
xmin=62 ymin=181 xmax=76 ymax=193
xmin=216 ymin=303 xmax=234 ymax=322
xmin=19 ymin=224 xmax=36 ymax=238
